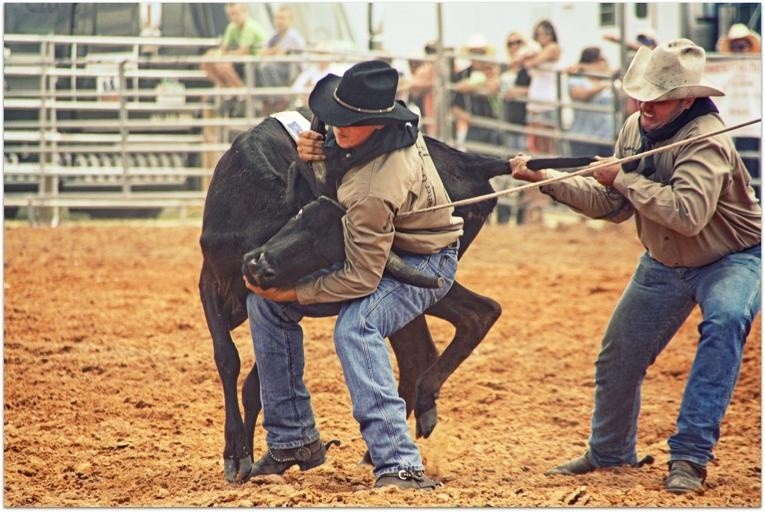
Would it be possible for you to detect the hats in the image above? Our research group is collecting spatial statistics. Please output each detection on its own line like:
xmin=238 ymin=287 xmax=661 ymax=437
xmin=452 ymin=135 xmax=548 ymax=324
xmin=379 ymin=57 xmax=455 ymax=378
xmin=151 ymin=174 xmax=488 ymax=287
xmin=460 ymin=33 xmax=496 ymax=59
xmin=309 ymin=61 xmax=419 ymax=127
xmin=623 ymin=38 xmax=725 ymax=102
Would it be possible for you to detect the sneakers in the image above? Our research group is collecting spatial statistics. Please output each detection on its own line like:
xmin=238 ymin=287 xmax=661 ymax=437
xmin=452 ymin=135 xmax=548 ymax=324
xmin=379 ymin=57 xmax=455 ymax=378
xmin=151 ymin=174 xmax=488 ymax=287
xmin=374 ymin=471 xmax=435 ymax=493
xmin=247 ymin=439 xmax=326 ymax=478
xmin=665 ymin=461 xmax=708 ymax=494
xmin=548 ymin=451 xmax=595 ymax=476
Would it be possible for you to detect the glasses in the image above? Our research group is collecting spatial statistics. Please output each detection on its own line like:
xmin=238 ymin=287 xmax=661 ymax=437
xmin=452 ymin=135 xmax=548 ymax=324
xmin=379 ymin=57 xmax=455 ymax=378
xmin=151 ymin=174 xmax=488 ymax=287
xmin=507 ymin=40 xmax=521 ymax=47
xmin=729 ymin=44 xmax=746 ymax=51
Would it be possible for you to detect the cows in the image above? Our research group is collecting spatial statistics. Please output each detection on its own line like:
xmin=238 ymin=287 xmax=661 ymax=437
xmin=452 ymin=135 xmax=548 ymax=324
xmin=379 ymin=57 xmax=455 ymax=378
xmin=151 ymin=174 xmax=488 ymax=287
xmin=199 ymin=110 xmax=642 ymax=484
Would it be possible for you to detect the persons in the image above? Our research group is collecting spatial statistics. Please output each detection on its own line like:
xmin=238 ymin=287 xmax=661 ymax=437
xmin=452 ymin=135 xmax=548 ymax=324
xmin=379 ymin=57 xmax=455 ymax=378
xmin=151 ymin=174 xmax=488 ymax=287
xmin=507 ymin=30 xmax=532 ymax=126
xmin=513 ymin=21 xmax=560 ymax=158
xmin=257 ymin=5 xmax=306 ymax=111
xmin=508 ymin=38 xmax=764 ymax=496
xmin=244 ymin=61 xmax=465 ymax=491
xmin=452 ymin=45 xmax=503 ymax=147
xmin=603 ymin=32 xmax=657 ymax=51
xmin=285 ymin=46 xmax=343 ymax=102
xmin=568 ymin=46 xmax=621 ymax=160
xmin=409 ymin=40 xmax=450 ymax=136
xmin=203 ymin=2 xmax=265 ymax=103
